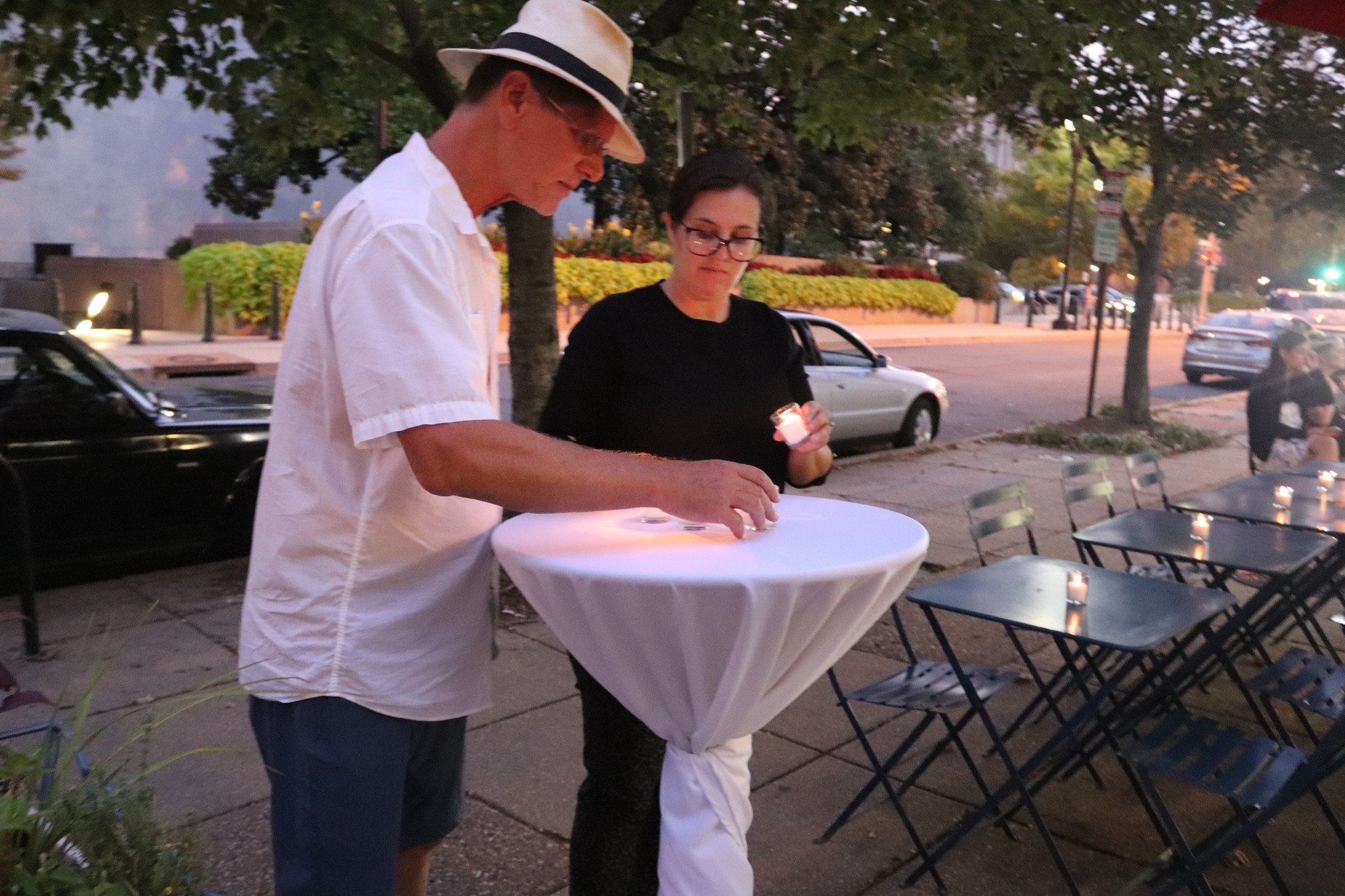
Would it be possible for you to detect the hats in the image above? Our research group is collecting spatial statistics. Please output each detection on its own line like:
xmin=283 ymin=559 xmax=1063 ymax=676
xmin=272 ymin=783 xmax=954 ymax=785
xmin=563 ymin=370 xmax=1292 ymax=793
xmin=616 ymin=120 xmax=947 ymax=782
xmin=437 ymin=0 xmax=648 ymax=166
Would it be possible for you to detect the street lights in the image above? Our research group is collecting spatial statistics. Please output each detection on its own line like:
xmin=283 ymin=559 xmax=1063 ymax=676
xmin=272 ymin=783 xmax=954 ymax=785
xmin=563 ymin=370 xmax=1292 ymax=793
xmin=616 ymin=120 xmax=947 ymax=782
xmin=1051 ymin=111 xmax=1095 ymax=329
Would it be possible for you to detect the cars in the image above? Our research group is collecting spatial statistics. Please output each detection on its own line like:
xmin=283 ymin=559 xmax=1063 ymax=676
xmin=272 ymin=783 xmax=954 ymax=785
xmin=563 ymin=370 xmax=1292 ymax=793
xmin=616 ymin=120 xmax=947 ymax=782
xmin=775 ymin=310 xmax=950 ymax=456
xmin=996 ymin=271 xmax=1135 ymax=316
xmin=1180 ymin=307 xmax=1345 ymax=388
xmin=1 ymin=307 xmax=279 ymax=562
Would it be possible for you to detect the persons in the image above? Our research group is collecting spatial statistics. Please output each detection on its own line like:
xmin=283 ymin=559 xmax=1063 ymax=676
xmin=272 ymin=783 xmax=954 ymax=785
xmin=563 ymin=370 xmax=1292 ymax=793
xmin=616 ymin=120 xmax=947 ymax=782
xmin=1247 ymin=330 xmax=1345 ymax=473
xmin=535 ymin=151 xmax=835 ymax=895
xmin=237 ymin=0 xmax=780 ymax=896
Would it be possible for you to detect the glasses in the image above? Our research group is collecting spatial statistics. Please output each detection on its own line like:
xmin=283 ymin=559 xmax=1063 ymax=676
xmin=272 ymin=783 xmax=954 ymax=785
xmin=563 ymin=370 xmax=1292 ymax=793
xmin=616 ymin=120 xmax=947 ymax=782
xmin=531 ymin=80 xmax=612 ymax=157
xmin=677 ymin=218 xmax=766 ymax=262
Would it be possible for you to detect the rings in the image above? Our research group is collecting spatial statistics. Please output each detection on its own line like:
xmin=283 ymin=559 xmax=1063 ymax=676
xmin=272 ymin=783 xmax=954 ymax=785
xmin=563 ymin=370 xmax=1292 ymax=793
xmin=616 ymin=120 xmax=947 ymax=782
xmin=826 ymin=421 xmax=836 ymax=433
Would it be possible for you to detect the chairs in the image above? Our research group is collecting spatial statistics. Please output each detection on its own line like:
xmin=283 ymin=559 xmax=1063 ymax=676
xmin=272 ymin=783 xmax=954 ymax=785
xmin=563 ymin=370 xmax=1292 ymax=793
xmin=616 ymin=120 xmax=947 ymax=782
xmin=815 ymin=452 xmax=1345 ymax=896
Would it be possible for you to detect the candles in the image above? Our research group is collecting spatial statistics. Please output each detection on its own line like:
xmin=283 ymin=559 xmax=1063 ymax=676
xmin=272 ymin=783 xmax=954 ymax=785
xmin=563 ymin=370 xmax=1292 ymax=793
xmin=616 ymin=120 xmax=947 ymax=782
xmin=772 ymin=402 xmax=813 ymax=446
xmin=1274 ymin=485 xmax=1294 ymax=509
xmin=1066 ymin=571 xmax=1088 ymax=605
xmin=1190 ymin=512 xmax=1211 ymax=541
xmin=1317 ymin=470 xmax=1336 ymax=487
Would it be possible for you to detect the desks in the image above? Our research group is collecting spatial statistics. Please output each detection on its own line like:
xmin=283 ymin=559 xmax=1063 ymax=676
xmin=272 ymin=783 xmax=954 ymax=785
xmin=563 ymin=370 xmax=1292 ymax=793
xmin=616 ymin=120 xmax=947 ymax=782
xmin=1285 ymin=459 xmax=1345 ymax=480
xmin=491 ymin=495 xmax=930 ymax=896
xmin=1225 ymin=471 xmax=1345 ymax=502
xmin=1167 ymin=485 xmax=1345 ymax=535
xmin=1071 ymin=508 xmax=1338 ymax=584
xmin=904 ymin=553 xmax=1239 ymax=896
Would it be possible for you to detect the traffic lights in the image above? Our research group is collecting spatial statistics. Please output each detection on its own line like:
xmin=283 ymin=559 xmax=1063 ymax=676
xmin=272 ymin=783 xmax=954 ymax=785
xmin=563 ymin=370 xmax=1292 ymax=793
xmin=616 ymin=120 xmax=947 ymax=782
xmin=1325 ymin=269 xmax=1339 ymax=282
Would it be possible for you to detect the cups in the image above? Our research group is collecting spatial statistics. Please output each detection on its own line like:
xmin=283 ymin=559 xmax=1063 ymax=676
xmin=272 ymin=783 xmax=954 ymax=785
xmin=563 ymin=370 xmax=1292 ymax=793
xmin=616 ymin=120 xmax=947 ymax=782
xmin=769 ymin=402 xmax=813 ymax=449
xmin=1067 ymin=572 xmax=1089 ymax=605
xmin=742 ymin=484 xmax=779 ymax=531
xmin=1316 ymin=469 xmax=1338 ymax=492
xmin=1272 ymin=485 xmax=1295 ymax=510
xmin=1189 ymin=512 xmax=1214 ymax=541
